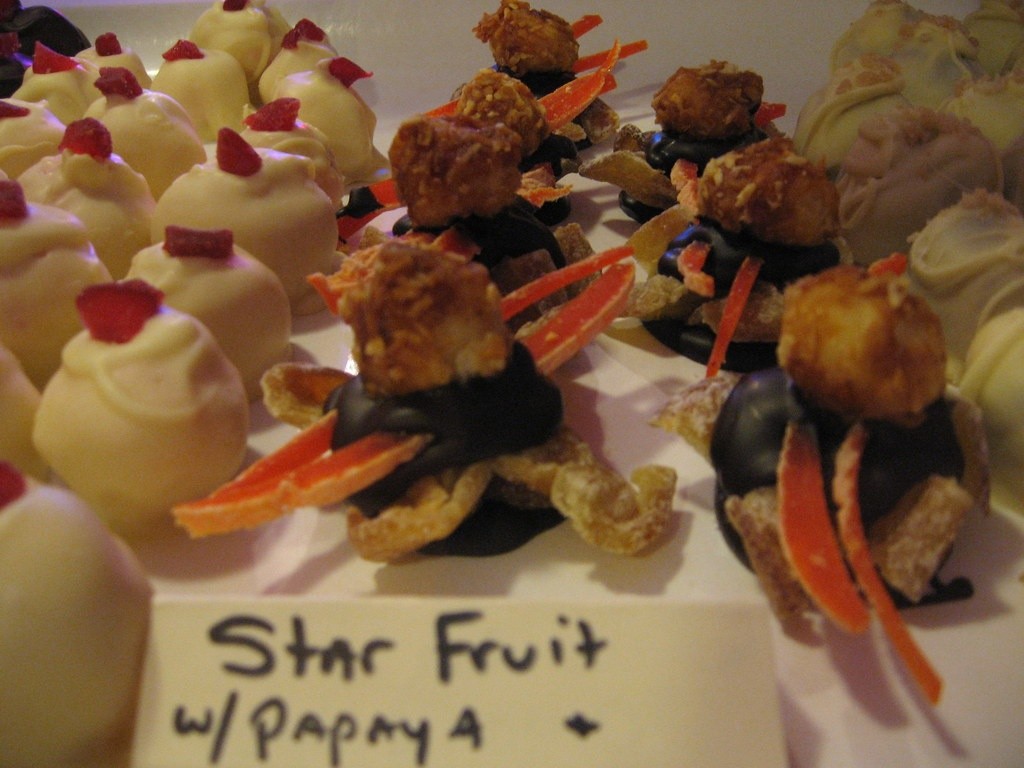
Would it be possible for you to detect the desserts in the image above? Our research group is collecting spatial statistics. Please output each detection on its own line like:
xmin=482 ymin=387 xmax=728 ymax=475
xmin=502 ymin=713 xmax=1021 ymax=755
xmin=0 ymin=0 xmax=378 ymax=768
xmin=792 ymin=0 xmax=1024 ymax=524
